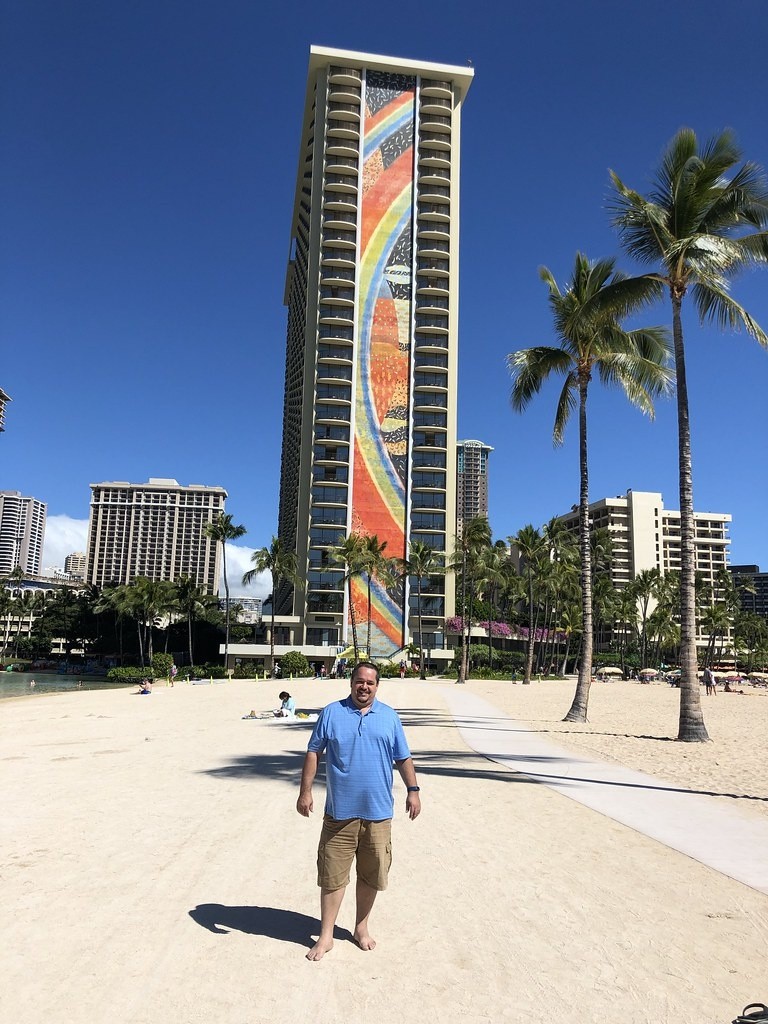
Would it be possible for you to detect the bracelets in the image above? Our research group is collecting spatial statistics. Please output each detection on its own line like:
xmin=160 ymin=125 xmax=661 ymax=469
xmin=407 ymin=784 xmax=420 ymax=794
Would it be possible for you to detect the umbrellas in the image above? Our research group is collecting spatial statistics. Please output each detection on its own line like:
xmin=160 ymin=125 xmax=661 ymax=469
xmin=639 ymin=668 xmax=768 ymax=682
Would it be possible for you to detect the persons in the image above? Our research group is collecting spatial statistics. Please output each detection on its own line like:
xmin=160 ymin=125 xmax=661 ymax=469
xmin=274 ymin=663 xmax=282 ymax=679
xmin=137 ymin=679 xmax=152 ymax=694
xmin=511 ymin=667 xmax=517 ymax=684
xmin=274 ymin=691 xmax=295 ymax=718
xmin=412 ymin=661 xmax=421 ymax=678
xmin=169 ymin=665 xmax=178 ymax=687
xmin=297 ymin=657 xmax=425 ymax=962
xmin=30 ymin=680 xmax=36 ymax=688
xmin=724 ymin=682 xmax=743 ymax=692
xmin=319 ymin=665 xmax=326 ymax=678
xmin=77 ymin=680 xmax=85 ymax=688
xmin=335 ymin=662 xmax=347 ymax=679
xmin=590 ymin=672 xmax=684 ymax=687
xmin=704 ymin=665 xmax=717 ymax=696
xmin=400 ymin=659 xmax=407 ymax=679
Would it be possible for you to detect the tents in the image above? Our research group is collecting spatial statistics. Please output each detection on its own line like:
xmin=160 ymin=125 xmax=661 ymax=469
xmin=596 ymin=667 xmax=623 ymax=680
